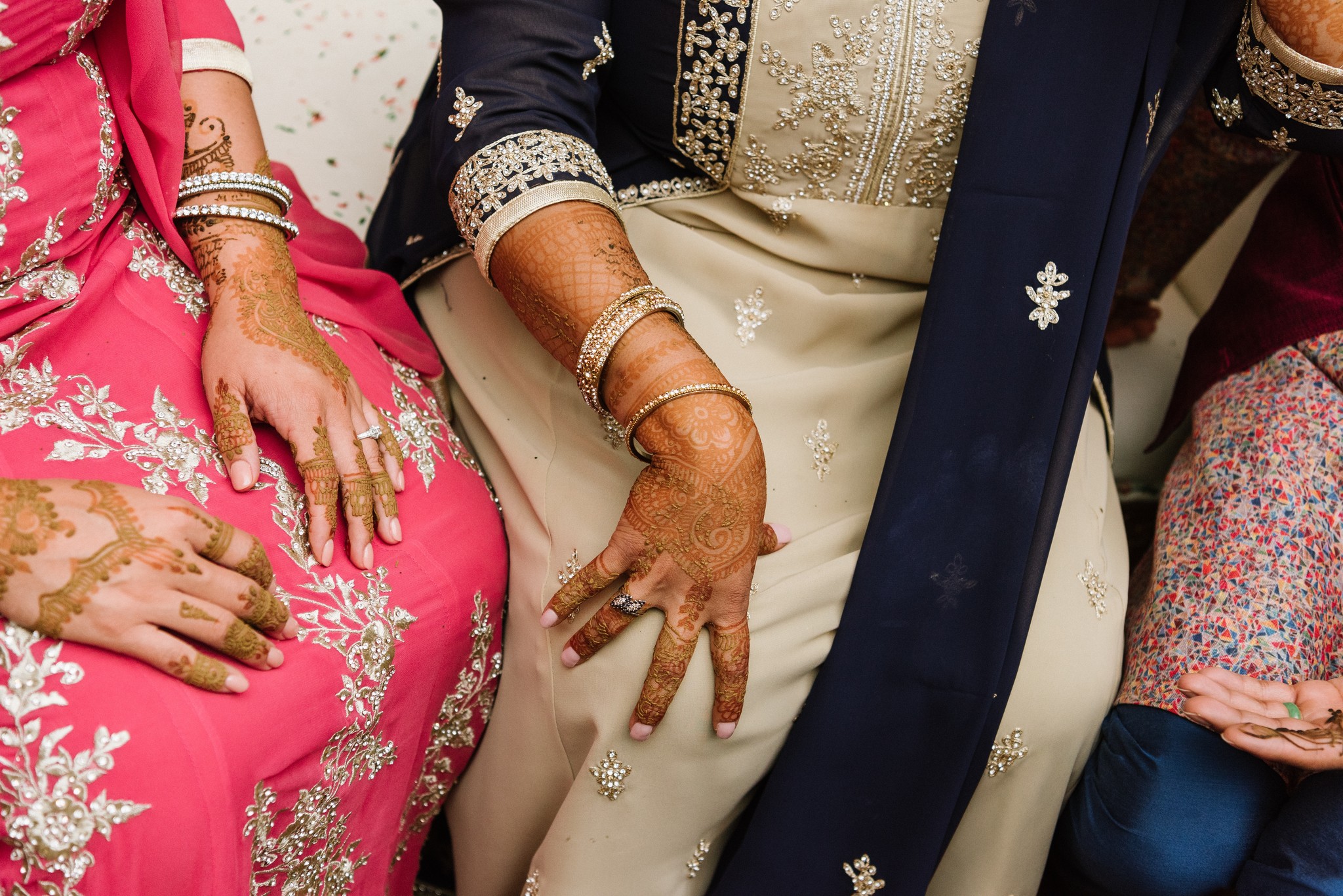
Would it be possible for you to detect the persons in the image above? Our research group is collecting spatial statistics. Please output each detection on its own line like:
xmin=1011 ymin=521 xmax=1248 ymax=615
xmin=1035 ymin=123 xmax=1343 ymax=896
xmin=0 ymin=0 xmax=512 ymax=896
xmin=361 ymin=1 xmax=1342 ymax=895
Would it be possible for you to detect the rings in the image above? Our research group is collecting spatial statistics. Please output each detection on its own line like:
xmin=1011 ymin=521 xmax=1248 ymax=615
xmin=354 ymin=421 xmax=385 ymax=441
xmin=611 ymin=583 xmax=650 ymax=617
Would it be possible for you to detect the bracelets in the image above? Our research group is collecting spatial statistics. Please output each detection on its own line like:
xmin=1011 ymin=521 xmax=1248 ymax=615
xmin=565 ymin=284 xmax=685 ymax=449
xmin=622 ymin=378 xmax=752 ymax=462
xmin=171 ymin=166 xmax=300 ymax=239
